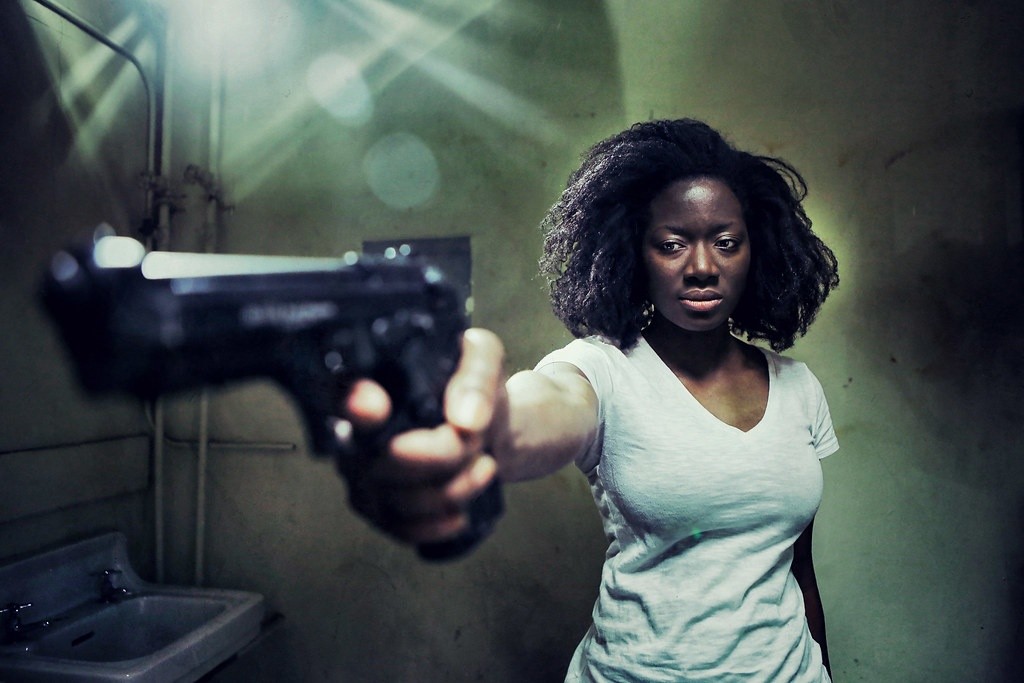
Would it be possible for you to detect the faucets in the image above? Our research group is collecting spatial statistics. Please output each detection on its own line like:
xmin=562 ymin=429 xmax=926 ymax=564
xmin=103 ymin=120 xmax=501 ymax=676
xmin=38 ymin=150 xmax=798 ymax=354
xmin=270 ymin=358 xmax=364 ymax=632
xmin=0 ymin=601 xmax=56 ymax=645
xmin=97 ymin=565 xmax=138 ymax=604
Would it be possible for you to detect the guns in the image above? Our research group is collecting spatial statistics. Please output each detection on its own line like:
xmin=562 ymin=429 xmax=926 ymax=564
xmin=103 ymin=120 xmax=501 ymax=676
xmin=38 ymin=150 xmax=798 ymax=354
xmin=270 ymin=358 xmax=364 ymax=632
xmin=30 ymin=236 xmax=511 ymax=566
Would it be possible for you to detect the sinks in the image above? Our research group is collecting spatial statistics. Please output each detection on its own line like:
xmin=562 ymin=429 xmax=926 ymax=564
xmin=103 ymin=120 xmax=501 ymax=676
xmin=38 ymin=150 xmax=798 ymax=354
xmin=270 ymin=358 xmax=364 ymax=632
xmin=0 ymin=585 xmax=268 ymax=683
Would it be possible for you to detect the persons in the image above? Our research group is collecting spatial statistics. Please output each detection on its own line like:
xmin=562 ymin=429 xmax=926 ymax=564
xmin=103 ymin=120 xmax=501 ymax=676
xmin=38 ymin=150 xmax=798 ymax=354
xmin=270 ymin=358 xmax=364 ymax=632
xmin=337 ymin=118 xmax=838 ymax=683
